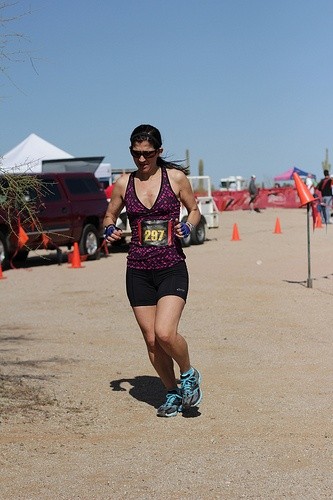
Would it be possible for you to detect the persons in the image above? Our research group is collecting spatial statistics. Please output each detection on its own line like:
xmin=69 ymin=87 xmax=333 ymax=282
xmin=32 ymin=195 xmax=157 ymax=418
xmin=103 ymin=124 xmax=202 ymax=417
xmin=318 ymin=169 xmax=333 ymax=224
xmin=305 ymin=174 xmax=314 ymax=195
xmin=249 ymin=175 xmax=257 ymax=210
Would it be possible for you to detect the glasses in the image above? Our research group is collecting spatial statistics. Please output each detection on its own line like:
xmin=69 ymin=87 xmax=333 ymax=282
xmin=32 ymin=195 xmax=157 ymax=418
xmin=128 ymin=146 xmax=158 ymax=158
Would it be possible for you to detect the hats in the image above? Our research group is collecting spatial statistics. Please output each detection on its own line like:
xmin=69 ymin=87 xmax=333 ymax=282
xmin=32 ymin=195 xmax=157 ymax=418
xmin=251 ymin=175 xmax=256 ymax=179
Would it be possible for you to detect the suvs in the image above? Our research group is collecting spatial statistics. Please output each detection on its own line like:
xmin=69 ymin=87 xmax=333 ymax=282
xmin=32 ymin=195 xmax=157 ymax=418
xmin=0 ymin=171 xmax=109 ymax=271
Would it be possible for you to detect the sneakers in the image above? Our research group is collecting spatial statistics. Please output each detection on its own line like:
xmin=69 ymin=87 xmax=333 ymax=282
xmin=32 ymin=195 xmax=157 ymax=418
xmin=156 ymin=385 xmax=183 ymax=417
xmin=174 ymin=366 xmax=203 ymax=411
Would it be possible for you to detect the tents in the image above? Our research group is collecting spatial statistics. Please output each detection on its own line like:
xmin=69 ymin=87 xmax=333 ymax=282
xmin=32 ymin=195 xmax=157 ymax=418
xmin=274 ymin=167 xmax=316 ymax=181
xmin=0 ymin=133 xmax=112 ymax=186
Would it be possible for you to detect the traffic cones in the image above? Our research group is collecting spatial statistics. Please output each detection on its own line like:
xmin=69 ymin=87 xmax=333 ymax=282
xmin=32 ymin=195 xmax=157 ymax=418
xmin=272 ymin=217 xmax=283 ymax=234
xmin=315 ymin=212 xmax=324 ymax=228
xmin=230 ymin=223 xmax=241 ymax=241
xmin=0 ymin=261 xmax=7 ymax=280
xmin=68 ymin=241 xmax=85 ymax=268
xmin=293 ymin=171 xmax=319 ymax=208
